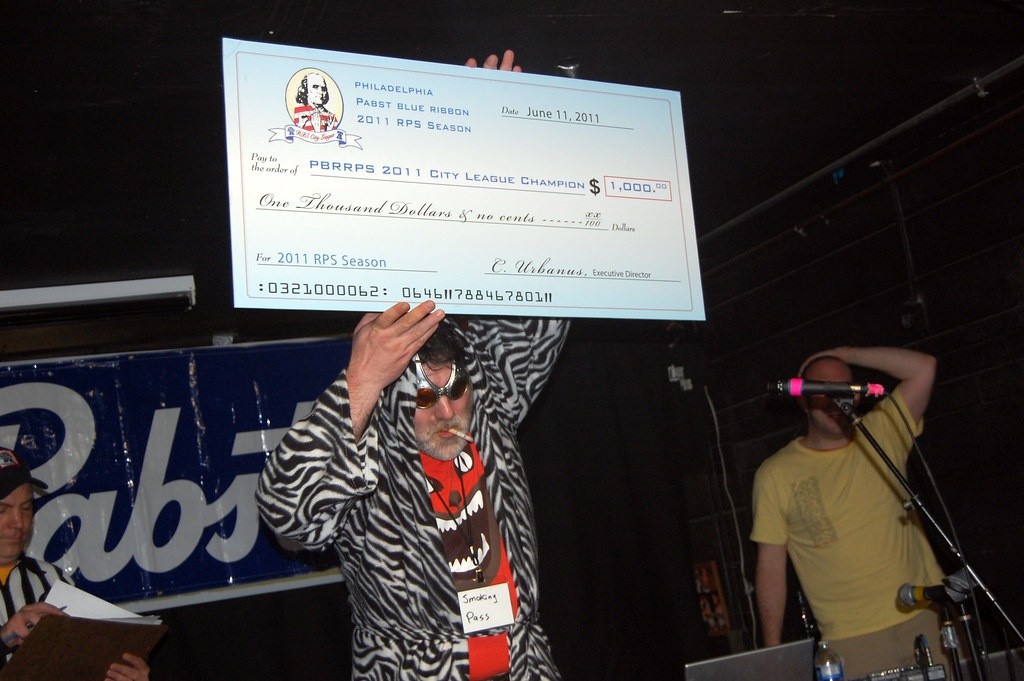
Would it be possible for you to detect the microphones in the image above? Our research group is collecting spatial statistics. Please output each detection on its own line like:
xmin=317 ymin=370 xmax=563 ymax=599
xmin=768 ymin=377 xmax=885 ymax=397
xmin=898 ymin=583 xmax=973 ymax=606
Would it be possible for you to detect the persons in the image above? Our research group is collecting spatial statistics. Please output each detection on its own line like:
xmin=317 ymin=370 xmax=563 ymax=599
xmin=255 ymin=50 xmax=574 ymax=681
xmin=0 ymin=446 xmax=151 ymax=681
xmin=749 ymin=346 xmax=972 ymax=681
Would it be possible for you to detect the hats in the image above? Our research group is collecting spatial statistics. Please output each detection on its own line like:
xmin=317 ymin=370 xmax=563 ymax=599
xmin=0 ymin=446 xmax=48 ymax=500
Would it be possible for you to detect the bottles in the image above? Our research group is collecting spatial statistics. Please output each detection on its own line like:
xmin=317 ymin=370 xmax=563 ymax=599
xmin=814 ymin=641 xmax=846 ymax=681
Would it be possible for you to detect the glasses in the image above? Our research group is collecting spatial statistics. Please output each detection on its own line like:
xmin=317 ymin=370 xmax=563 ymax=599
xmin=412 ymin=354 xmax=469 ymax=409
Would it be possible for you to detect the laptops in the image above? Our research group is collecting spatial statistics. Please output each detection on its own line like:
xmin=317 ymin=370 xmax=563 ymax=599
xmin=685 ymin=638 xmax=817 ymax=681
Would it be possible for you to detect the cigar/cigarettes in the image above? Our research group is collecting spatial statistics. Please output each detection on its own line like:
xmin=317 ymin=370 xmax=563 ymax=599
xmin=449 ymin=429 xmax=474 ymax=442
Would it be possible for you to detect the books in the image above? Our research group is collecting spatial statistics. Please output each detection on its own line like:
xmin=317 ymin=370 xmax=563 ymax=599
xmin=0 ymin=578 xmax=167 ymax=681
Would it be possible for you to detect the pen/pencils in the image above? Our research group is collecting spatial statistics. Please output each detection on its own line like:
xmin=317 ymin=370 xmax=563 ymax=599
xmin=3 ymin=605 xmax=67 ymax=642
